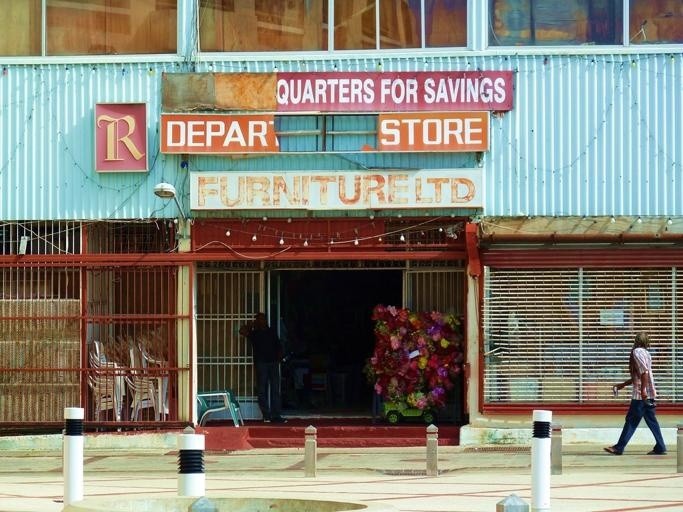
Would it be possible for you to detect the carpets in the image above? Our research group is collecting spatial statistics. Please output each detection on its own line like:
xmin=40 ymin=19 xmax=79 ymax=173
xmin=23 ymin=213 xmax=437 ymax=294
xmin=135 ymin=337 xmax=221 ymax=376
xmin=84 ymin=418 xmax=458 ymax=451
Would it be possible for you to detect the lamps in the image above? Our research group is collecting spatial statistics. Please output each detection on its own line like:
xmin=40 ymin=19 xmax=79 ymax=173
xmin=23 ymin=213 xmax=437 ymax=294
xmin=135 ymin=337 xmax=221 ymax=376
xmin=154 ymin=183 xmax=192 ymax=225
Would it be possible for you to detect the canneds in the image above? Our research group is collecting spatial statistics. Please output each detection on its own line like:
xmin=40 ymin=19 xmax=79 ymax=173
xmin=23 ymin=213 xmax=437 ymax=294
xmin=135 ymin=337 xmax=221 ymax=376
xmin=613 ymin=386 xmax=618 ymax=396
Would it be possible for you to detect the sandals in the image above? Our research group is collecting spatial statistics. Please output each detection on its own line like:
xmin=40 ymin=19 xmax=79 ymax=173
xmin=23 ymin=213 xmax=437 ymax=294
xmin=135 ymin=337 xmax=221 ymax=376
xmin=603 ymin=445 xmax=623 ymax=455
xmin=646 ymin=449 xmax=668 ymax=455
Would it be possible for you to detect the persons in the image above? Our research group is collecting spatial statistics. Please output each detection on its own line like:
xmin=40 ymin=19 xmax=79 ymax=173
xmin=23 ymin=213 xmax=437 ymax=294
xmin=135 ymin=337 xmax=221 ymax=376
xmin=603 ymin=331 xmax=668 ymax=455
xmin=239 ymin=312 xmax=288 ymax=424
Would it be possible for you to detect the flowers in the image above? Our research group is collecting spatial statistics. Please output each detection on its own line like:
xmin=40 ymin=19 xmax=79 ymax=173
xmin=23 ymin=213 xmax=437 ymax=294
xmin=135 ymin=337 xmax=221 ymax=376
xmin=358 ymin=302 xmax=461 ymax=411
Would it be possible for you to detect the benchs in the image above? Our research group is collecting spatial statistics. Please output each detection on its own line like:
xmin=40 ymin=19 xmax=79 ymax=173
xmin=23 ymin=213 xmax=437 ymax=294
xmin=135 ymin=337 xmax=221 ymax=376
xmin=197 ymin=396 xmax=264 ymax=420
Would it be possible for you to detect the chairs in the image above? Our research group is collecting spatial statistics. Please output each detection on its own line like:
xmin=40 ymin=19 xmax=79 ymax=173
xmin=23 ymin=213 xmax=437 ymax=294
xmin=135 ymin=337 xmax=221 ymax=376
xmin=87 ymin=338 xmax=170 ymax=432
xmin=196 ymin=387 xmax=244 ymax=427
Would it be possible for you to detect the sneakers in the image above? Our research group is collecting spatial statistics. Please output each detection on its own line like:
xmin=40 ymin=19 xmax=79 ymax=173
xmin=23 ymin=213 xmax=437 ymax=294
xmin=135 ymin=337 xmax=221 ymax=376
xmin=263 ymin=416 xmax=288 ymax=423
